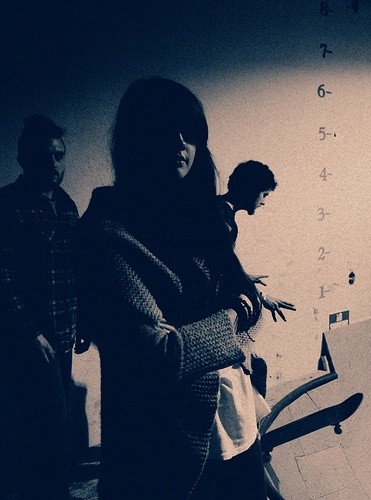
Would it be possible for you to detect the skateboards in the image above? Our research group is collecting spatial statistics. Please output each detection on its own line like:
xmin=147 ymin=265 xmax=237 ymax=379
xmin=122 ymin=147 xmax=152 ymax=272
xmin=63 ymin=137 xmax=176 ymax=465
xmin=259 ymin=391 xmax=364 ymax=462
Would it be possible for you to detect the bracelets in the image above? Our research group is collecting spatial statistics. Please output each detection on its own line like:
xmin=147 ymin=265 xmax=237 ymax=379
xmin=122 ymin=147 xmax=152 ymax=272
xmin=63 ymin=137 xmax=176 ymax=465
xmin=229 ymin=297 xmax=252 ymax=331
xmin=260 ymin=292 xmax=266 ymax=302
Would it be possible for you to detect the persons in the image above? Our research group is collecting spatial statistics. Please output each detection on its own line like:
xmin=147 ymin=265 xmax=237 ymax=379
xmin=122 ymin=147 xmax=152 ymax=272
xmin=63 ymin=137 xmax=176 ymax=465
xmin=216 ymin=161 xmax=297 ymax=322
xmin=0 ymin=117 xmax=91 ymax=497
xmin=67 ymin=77 xmax=269 ymax=499
